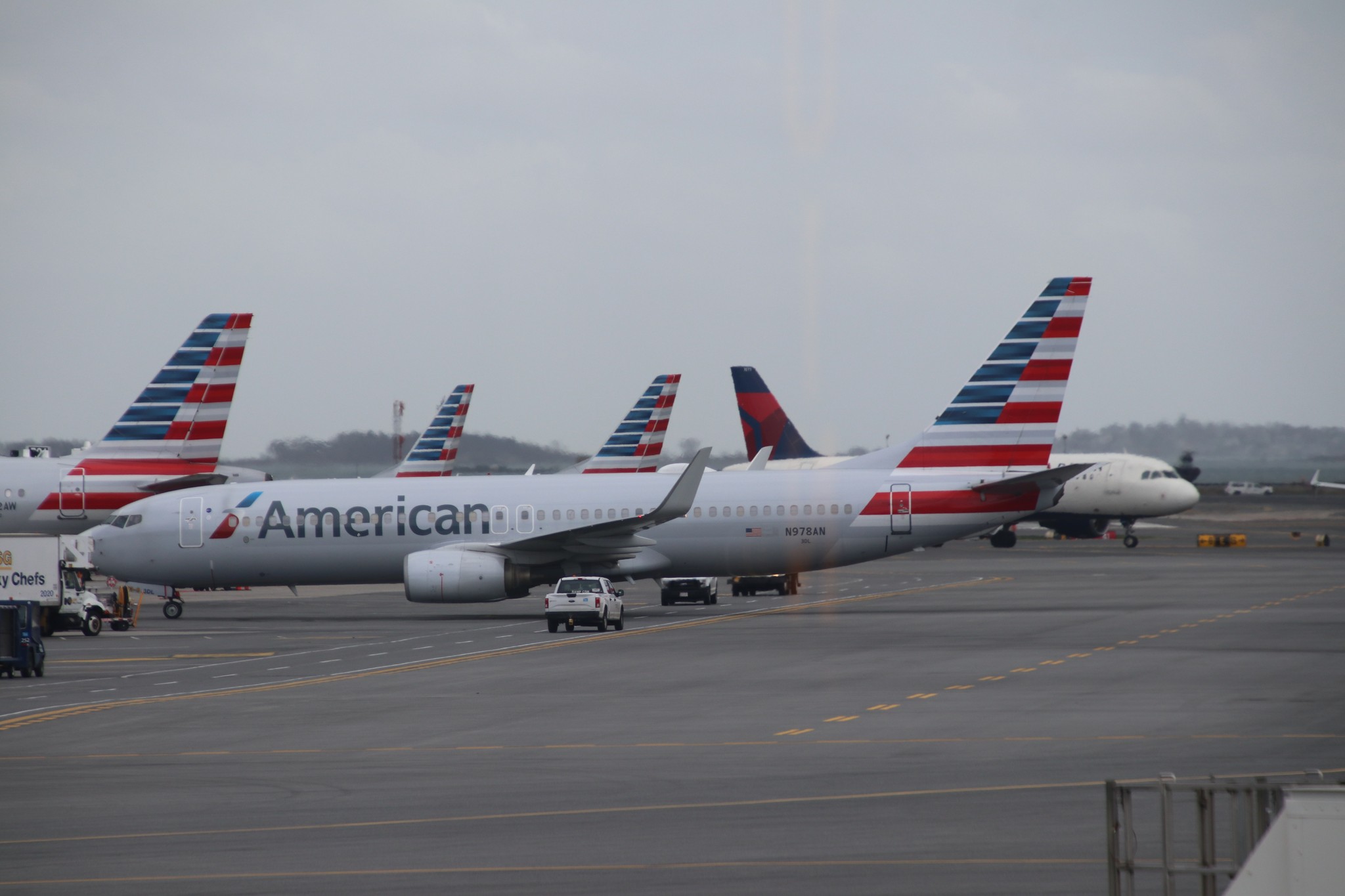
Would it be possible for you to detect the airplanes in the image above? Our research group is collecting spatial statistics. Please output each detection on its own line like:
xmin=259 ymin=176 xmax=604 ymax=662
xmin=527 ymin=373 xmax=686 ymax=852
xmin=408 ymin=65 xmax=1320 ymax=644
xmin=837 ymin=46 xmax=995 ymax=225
xmin=73 ymin=273 xmax=1110 ymax=620
xmin=721 ymin=364 xmax=1202 ymax=550
xmin=0 ymin=309 xmax=280 ymax=534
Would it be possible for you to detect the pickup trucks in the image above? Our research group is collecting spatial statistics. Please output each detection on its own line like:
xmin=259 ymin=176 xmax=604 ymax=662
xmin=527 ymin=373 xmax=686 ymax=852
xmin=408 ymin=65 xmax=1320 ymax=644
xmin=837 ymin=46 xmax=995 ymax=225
xmin=544 ymin=576 xmax=625 ymax=633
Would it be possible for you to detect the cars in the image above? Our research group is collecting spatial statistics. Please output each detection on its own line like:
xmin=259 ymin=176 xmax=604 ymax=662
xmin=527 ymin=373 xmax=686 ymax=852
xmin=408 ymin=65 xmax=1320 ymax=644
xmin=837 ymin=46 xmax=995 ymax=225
xmin=658 ymin=575 xmax=720 ymax=605
xmin=1222 ymin=481 xmax=1275 ymax=497
xmin=725 ymin=575 xmax=801 ymax=596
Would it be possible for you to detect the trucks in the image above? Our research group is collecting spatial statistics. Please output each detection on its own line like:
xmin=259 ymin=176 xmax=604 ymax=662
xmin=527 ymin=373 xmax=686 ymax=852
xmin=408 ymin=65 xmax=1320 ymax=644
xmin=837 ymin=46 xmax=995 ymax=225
xmin=2 ymin=537 xmax=108 ymax=637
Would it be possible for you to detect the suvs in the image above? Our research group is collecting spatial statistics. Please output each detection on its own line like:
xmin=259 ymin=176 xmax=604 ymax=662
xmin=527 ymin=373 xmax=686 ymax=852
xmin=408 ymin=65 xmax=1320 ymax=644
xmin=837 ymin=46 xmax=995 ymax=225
xmin=0 ymin=597 xmax=47 ymax=678
